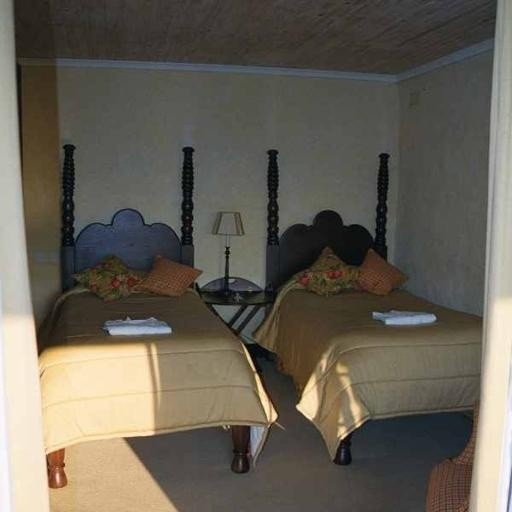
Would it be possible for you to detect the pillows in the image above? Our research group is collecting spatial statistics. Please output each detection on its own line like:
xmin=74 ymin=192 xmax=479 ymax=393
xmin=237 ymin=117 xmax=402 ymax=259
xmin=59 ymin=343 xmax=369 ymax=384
xmin=72 ymin=256 xmax=147 ymax=304
xmin=138 ymin=254 xmax=203 ymax=297
xmin=298 ymin=247 xmax=358 ymax=298
xmin=353 ymin=249 xmax=409 ymax=296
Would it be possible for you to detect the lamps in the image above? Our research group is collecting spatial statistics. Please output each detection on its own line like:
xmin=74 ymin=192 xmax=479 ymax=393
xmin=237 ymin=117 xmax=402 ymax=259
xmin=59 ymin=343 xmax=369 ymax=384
xmin=212 ymin=211 xmax=245 ymax=294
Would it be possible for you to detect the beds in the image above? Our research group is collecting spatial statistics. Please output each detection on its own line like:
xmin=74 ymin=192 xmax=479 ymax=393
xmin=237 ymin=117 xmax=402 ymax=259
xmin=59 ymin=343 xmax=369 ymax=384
xmin=251 ymin=149 xmax=482 ymax=464
xmin=39 ymin=144 xmax=279 ymax=488
